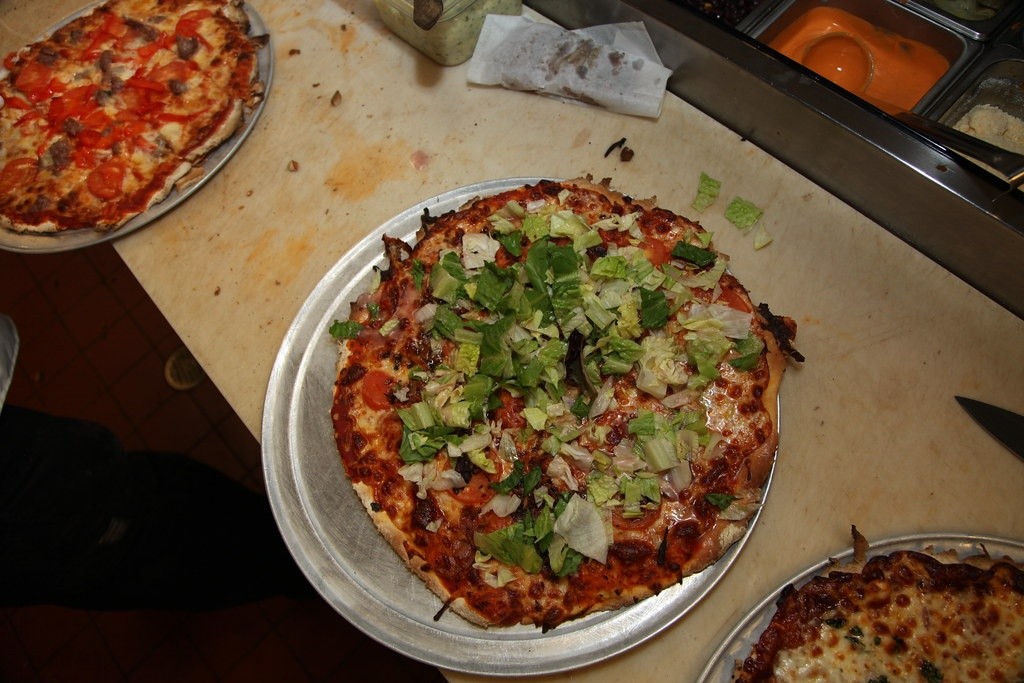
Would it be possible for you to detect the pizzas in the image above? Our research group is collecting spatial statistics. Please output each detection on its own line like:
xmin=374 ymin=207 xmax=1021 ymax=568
xmin=329 ymin=176 xmax=806 ymax=636
xmin=1 ymin=0 xmax=272 ymax=237
xmin=726 ymin=521 xmax=1024 ymax=683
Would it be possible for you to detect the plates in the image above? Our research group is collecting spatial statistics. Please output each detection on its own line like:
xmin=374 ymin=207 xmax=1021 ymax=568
xmin=262 ymin=178 xmax=782 ymax=678
xmin=0 ymin=0 xmax=276 ymax=253
xmin=685 ymin=530 xmax=1023 ymax=682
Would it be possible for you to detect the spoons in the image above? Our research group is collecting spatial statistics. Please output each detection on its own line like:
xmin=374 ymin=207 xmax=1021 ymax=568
xmin=797 ymin=30 xmax=1024 ymax=182
